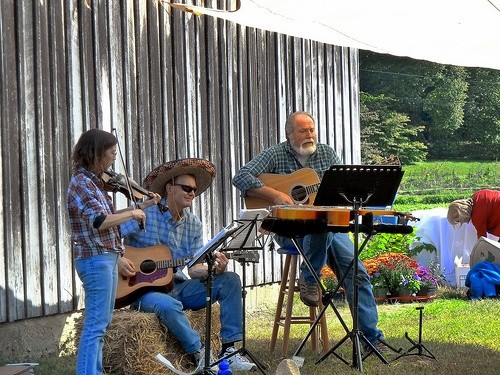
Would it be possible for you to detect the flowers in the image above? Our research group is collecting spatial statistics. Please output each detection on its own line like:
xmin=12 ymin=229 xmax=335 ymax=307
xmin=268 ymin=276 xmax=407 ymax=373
xmin=321 ymin=252 xmax=436 ymax=287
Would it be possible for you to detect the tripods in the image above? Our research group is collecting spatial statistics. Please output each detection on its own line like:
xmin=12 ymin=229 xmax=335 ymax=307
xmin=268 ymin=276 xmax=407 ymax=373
xmin=208 ymin=212 xmax=277 ymax=375
xmin=394 ymin=306 xmax=439 ymax=364
xmin=312 ymin=164 xmax=405 ymax=372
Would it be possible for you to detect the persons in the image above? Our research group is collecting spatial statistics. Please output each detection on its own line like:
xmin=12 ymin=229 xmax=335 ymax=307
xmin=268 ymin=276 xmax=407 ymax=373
xmin=231 ymin=111 xmax=391 ymax=354
xmin=118 ymin=159 xmax=257 ymax=374
xmin=447 ymin=189 xmax=500 ymax=243
xmin=68 ymin=129 xmax=161 ymax=375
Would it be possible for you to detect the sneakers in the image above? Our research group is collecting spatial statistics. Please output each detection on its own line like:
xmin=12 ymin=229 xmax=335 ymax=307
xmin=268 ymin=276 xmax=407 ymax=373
xmin=360 ymin=340 xmax=391 ymax=356
xmin=298 ymin=273 xmax=319 ymax=307
xmin=193 ymin=342 xmax=220 ymax=375
xmin=222 ymin=345 xmax=257 ymax=371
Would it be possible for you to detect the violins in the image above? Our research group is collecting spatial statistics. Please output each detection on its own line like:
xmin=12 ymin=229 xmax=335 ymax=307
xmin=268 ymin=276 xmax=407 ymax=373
xmin=101 ymin=169 xmax=170 ymax=214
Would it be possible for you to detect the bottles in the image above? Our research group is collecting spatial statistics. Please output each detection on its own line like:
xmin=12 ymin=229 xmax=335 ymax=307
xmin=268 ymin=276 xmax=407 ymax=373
xmin=218 ymin=359 xmax=232 ymax=375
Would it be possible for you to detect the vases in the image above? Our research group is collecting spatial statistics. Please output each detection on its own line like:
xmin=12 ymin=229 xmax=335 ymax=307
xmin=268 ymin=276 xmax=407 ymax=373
xmin=422 ymin=288 xmax=434 ymax=303
xmin=334 ymin=291 xmax=344 ymax=301
xmin=374 ymin=288 xmax=387 ymax=298
xmin=399 ymin=287 xmax=412 ymax=303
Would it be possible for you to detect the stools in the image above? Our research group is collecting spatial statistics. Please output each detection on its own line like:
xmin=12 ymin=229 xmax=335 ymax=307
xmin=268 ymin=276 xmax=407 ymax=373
xmin=269 ymin=248 xmax=329 ymax=355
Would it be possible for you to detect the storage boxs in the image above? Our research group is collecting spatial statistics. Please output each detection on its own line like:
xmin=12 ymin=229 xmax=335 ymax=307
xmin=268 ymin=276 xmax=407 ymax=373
xmin=456 ymin=265 xmax=472 ymax=286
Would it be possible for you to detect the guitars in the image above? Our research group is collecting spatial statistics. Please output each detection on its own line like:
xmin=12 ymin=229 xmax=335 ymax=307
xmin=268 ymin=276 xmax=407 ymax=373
xmin=116 ymin=244 xmax=260 ymax=309
xmin=244 ymin=167 xmax=323 ymax=233
xmin=271 ymin=203 xmax=421 ymax=228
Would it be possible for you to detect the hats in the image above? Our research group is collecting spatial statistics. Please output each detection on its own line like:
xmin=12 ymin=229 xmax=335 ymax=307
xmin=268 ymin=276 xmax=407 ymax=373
xmin=142 ymin=157 xmax=217 ymax=196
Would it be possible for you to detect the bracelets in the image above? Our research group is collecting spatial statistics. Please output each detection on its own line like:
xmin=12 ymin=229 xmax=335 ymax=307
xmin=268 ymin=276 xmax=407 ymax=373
xmin=130 ymin=210 xmax=137 ymax=220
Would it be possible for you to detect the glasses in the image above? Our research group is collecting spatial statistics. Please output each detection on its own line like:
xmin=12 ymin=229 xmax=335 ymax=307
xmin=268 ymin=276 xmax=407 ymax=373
xmin=171 ymin=182 xmax=197 ymax=192
xmin=113 ymin=152 xmax=117 ymax=156
